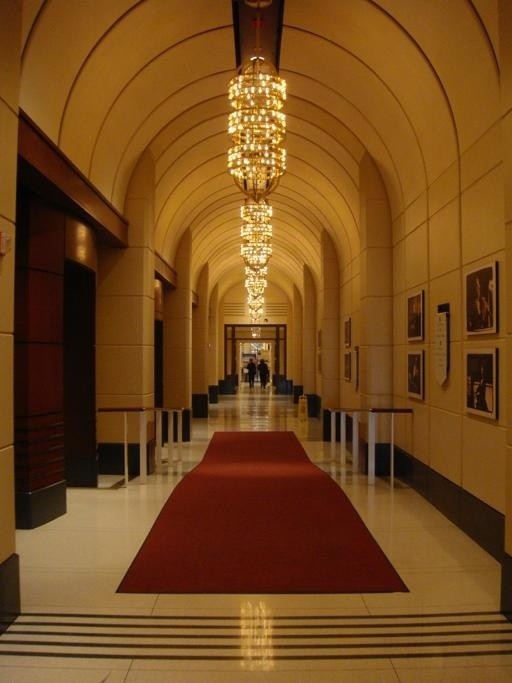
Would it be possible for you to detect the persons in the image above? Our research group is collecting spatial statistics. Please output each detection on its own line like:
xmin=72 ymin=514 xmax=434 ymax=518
xmin=247 ymin=357 xmax=256 ymax=388
xmin=474 ymin=360 xmax=489 ymax=412
xmin=257 ymin=358 xmax=270 ymax=388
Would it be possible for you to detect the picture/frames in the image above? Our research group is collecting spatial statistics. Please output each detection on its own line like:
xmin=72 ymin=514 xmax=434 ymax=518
xmin=464 ymin=260 xmax=498 ymax=421
xmin=343 ymin=317 xmax=351 ymax=382
xmin=407 ymin=289 xmax=424 ymax=401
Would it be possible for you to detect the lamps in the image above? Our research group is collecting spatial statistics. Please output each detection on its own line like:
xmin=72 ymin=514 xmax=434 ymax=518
xmin=226 ymin=0 xmax=287 ymax=339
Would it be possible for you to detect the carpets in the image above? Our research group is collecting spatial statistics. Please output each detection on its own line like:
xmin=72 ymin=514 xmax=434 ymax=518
xmin=116 ymin=431 xmax=410 ymax=594
